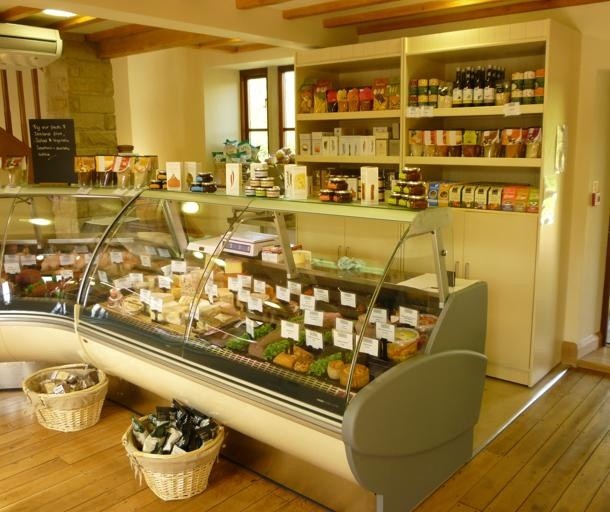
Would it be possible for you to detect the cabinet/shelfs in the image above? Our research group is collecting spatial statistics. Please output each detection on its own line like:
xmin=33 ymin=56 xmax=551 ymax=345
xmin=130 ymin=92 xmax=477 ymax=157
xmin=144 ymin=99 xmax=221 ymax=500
xmin=296 ymin=36 xmax=404 ymax=274
xmin=1 ymin=185 xmax=487 ymax=511
xmin=405 ymin=20 xmax=581 ymax=388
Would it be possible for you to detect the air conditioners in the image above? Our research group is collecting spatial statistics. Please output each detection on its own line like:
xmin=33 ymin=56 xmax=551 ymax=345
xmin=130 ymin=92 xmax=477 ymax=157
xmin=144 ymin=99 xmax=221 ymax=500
xmin=0 ymin=22 xmax=63 ymax=72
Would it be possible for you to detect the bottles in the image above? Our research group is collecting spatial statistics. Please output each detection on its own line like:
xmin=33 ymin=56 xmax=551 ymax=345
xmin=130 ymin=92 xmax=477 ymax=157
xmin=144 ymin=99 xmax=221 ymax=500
xmin=452 ymin=66 xmax=504 ymax=107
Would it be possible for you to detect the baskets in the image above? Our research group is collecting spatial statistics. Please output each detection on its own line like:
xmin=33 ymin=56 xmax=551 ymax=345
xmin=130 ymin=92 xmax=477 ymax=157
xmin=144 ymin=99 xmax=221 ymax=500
xmin=121 ymin=416 xmax=225 ymax=502
xmin=22 ymin=363 xmax=109 ymax=433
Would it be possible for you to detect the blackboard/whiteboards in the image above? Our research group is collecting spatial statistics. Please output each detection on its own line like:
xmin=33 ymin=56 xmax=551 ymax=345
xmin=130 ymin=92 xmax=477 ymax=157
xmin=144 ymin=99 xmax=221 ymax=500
xmin=29 ymin=119 xmax=78 ymax=183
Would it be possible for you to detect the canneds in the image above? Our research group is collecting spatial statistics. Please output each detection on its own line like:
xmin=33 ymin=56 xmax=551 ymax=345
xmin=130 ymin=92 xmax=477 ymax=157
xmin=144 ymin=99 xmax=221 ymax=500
xmin=496 ymin=67 xmax=545 ymax=105
xmin=319 ymin=178 xmax=352 ymax=202
xmin=388 ymin=167 xmax=428 ymax=208
xmin=356 ymin=177 xmax=384 ymax=202
xmin=191 ymin=173 xmax=218 ymax=193
xmin=246 ymin=168 xmax=280 ymax=199
xmin=407 ymin=78 xmax=444 ymax=107
xmin=147 ymin=170 xmax=168 ymax=190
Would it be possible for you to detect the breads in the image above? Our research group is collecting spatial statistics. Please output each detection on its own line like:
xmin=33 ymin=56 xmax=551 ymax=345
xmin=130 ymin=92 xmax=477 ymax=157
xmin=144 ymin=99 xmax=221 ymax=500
xmin=1 ymin=236 xmax=438 ymax=390
